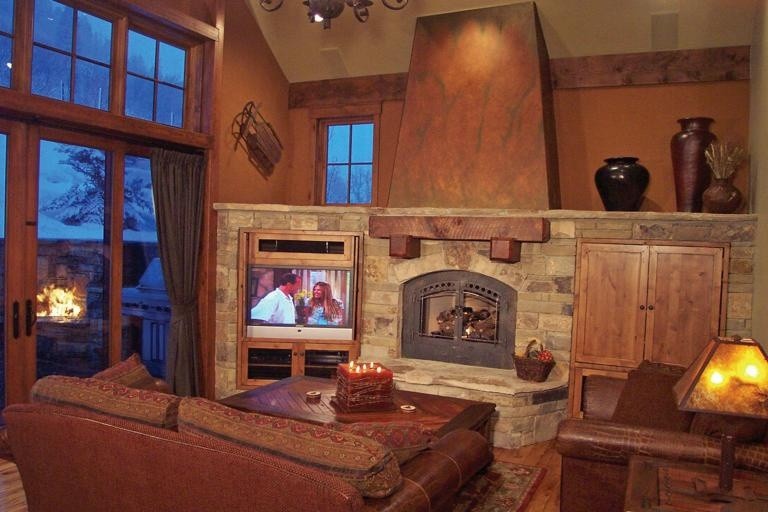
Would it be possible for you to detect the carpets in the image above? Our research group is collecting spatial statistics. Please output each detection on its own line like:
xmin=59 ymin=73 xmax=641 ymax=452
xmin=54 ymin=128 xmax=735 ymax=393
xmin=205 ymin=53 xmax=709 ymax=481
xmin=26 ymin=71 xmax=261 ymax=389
xmin=438 ymin=456 xmax=547 ymax=512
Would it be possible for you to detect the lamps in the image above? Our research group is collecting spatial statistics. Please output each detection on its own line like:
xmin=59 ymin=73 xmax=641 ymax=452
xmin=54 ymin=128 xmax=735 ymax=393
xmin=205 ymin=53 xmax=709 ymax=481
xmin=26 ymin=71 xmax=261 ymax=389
xmin=671 ymin=334 xmax=768 ymax=492
xmin=260 ymin=0 xmax=409 ymax=30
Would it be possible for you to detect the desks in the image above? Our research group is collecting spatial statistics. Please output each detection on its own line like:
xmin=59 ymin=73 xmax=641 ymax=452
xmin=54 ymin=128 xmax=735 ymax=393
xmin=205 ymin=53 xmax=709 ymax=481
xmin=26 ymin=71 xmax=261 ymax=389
xmin=623 ymin=456 xmax=767 ymax=512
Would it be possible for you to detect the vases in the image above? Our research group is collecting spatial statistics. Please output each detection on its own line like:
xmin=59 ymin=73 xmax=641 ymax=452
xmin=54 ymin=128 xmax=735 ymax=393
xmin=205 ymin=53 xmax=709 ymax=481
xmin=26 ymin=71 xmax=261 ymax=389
xmin=669 ymin=117 xmax=748 ymax=214
xmin=595 ymin=156 xmax=650 ymax=211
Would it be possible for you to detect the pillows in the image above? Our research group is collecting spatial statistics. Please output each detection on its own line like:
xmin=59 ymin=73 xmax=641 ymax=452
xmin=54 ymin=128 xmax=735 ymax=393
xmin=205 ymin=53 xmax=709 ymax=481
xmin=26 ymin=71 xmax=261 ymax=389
xmin=609 ymin=359 xmax=695 ymax=434
xmin=91 ymin=351 xmax=172 ymax=393
xmin=324 ymin=420 xmax=437 ymax=467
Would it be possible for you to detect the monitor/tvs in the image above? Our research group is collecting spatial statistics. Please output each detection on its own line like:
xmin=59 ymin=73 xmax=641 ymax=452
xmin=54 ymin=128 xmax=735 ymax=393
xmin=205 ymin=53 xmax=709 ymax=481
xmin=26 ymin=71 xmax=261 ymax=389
xmin=246 ymin=263 xmax=352 ymax=341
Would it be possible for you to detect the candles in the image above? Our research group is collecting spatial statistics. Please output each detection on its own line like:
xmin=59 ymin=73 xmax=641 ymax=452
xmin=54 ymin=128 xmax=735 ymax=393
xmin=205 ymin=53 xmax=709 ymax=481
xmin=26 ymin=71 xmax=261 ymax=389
xmin=349 ymin=360 xmax=382 ymax=374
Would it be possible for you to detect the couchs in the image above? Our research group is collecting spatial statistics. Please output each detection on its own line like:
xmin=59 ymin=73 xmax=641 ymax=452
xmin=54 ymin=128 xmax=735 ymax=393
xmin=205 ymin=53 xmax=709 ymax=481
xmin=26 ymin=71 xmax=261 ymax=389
xmin=2 ymin=374 xmax=494 ymax=512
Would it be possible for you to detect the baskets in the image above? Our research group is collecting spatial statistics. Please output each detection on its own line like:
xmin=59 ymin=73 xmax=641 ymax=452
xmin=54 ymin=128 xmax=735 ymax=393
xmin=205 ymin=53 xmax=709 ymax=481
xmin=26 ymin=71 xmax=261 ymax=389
xmin=512 ymin=338 xmax=556 ymax=382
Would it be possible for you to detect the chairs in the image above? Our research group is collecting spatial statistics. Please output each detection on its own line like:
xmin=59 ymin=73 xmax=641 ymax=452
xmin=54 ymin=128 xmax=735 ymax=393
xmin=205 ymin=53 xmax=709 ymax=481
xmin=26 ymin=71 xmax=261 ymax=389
xmin=553 ymin=374 xmax=768 ymax=512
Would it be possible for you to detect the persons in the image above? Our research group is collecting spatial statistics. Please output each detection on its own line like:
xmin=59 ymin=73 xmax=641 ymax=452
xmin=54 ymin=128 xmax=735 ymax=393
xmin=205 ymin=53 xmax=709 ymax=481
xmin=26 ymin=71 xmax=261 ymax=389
xmin=251 ymin=274 xmax=303 ymax=324
xmin=302 ymin=280 xmax=345 ymax=325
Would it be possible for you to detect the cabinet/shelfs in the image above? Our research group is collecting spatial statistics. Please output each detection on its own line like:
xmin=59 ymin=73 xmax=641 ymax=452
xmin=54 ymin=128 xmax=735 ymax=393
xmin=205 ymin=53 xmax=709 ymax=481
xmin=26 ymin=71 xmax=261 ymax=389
xmin=237 ymin=226 xmax=365 ymax=391
xmin=569 ymin=237 xmax=731 ymax=374
